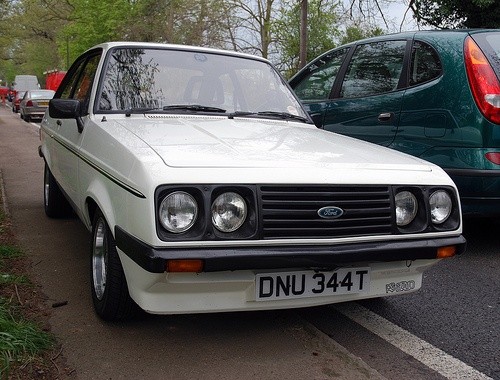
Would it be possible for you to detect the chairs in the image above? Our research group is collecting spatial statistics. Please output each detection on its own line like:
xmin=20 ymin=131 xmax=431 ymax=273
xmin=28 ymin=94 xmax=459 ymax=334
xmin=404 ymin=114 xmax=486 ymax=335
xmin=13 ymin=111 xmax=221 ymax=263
xmin=187 ymin=77 xmax=224 ymax=106
xmin=354 ymin=62 xmax=391 ymax=94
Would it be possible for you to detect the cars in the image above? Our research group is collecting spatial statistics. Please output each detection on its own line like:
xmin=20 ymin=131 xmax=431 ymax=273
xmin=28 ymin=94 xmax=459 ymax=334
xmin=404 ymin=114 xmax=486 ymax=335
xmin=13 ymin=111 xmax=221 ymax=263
xmin=0 ymin=75 xmax=39 ymax=113
xmin=18 ymin=90 xmax=57 ymax=121
xmin=260 ymin=28 xmax=500 ymax=236
xmin=37 ymin=41 xmax=468 ymax=326
